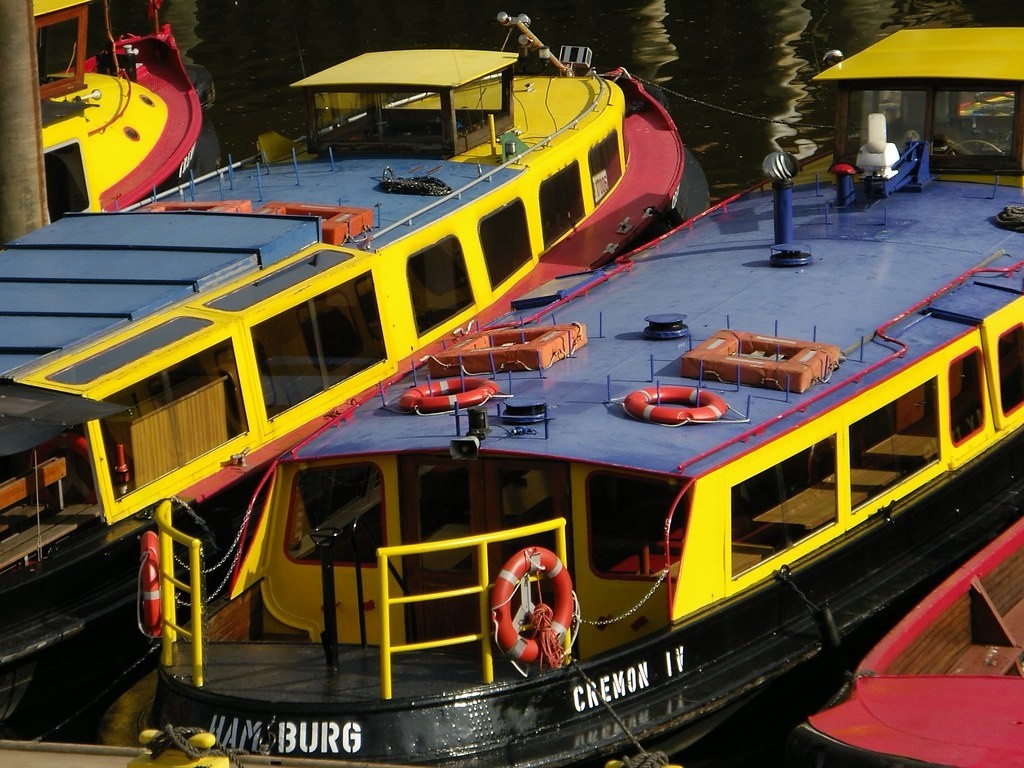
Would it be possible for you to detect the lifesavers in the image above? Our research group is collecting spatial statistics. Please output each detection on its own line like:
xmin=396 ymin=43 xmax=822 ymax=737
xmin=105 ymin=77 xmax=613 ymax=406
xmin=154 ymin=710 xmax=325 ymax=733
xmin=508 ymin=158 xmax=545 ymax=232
xmin=139 ymin=529 xmax=164 ymax=638
xmin=490 ymin=545 xmax=576 ymax=663
xmin=399 ymin=375 xmax=502 ymax=413
xmin=623 ymin=385 xmax=729 ymax=425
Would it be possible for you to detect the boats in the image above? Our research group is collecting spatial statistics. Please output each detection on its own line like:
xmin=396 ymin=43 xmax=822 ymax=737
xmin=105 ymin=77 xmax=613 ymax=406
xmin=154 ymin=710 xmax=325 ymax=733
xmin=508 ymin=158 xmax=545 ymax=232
xmin=0 ymin=0 xmax=1024 ymax=767
